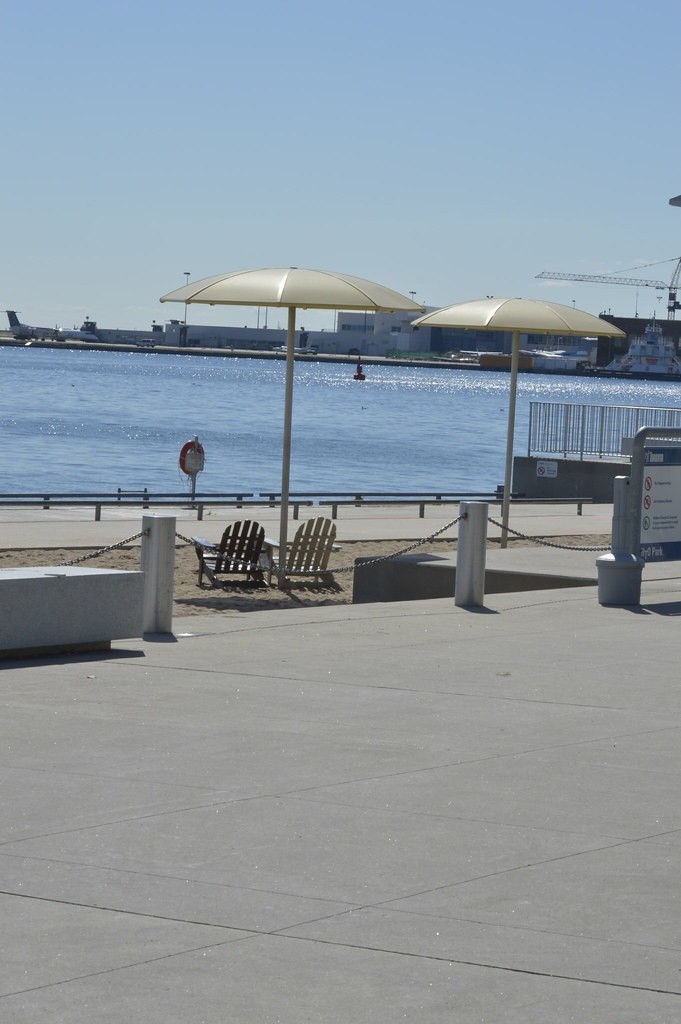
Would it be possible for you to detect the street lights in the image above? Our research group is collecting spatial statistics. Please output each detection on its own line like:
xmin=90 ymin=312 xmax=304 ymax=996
xmin=184 ymin=272 xmax=190 ymax=347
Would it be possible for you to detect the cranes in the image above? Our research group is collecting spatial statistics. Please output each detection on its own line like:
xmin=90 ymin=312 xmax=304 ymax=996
xmin=534 ymin=256 xmax=681 ymax=319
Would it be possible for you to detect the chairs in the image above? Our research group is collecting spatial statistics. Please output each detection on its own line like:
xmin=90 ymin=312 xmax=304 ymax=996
xmin=192 ymin=520 xmax=273 ymax=592
xmin=259 ymin=516 xmax=342 ymax=590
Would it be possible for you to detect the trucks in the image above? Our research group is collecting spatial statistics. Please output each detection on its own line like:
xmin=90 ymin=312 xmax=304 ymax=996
xmin=137 ymin=339 xmax=157 ymax=347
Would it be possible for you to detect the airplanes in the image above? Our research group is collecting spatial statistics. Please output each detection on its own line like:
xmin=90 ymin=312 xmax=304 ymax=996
xmin=0 ymin=310 xmax=98 ymax=342
xmin=433 ymin=348 xmax=566 ymax=363
xmin=272 ymin=341 xmax=317 ymax=355
